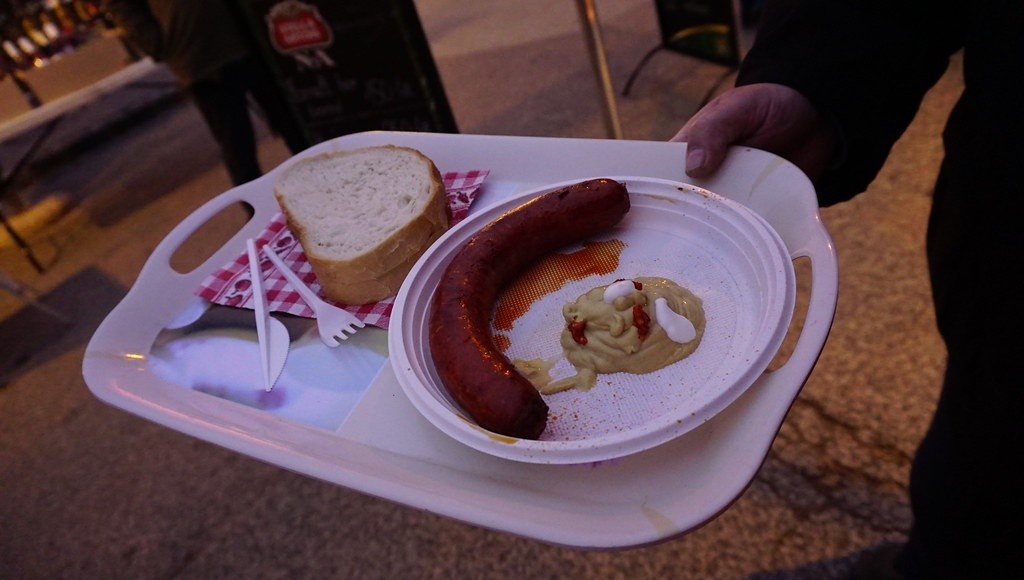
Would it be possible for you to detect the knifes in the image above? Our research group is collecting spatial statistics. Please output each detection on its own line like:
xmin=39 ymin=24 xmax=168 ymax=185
xmin=246 ymin=238 xmax=290 ymax=395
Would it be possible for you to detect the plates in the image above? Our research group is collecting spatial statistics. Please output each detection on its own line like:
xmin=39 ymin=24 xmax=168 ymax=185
xmin=388 ymin=176 xmax=797 ymax=465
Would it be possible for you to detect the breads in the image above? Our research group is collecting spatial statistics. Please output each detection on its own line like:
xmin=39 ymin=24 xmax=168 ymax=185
xmin=275 ymin=146 xmax=451 ymax=305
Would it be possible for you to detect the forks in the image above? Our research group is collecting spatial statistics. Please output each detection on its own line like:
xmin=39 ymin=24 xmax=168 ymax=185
xmin=261 ymin=243 xmax=366 ymax=348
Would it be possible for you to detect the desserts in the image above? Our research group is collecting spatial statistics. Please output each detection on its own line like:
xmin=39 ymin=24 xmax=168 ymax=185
xmin=562 ymin=280 xmax=705 ymax=371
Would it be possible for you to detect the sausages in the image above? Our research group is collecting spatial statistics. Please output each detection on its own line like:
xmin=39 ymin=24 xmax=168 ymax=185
xmin=427 ymin=179 xmax=630 ymax=441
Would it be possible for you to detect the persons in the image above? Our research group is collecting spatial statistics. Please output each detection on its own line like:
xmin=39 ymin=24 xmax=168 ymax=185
xmin=662 ymin=1 xmax=1024 ymax=580
xmin=101 ymin=0 xmax=309 ymax=230
